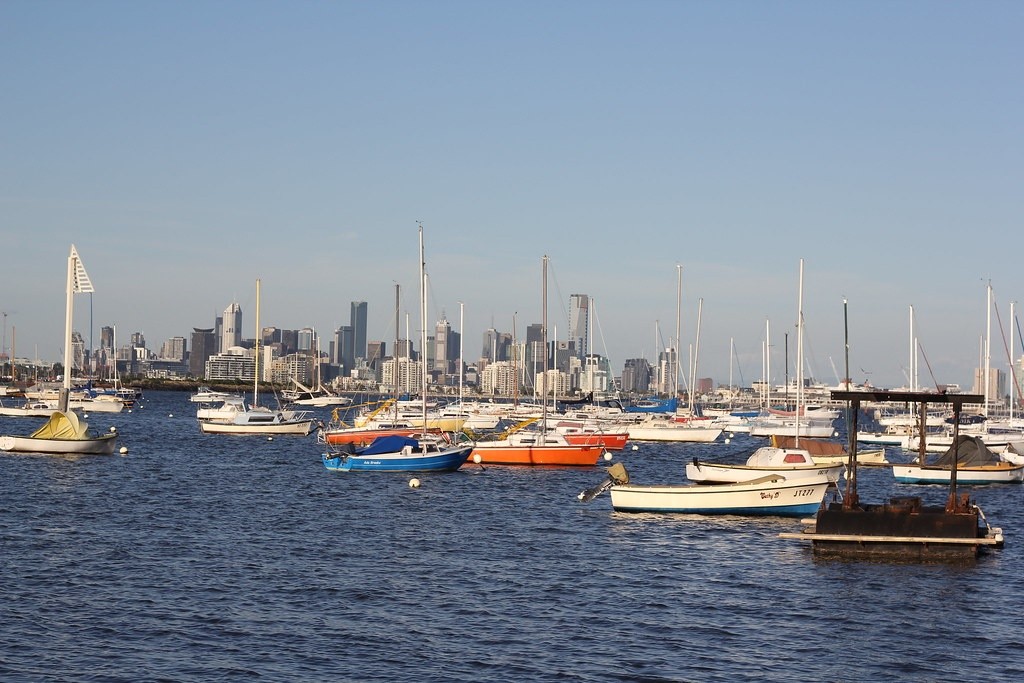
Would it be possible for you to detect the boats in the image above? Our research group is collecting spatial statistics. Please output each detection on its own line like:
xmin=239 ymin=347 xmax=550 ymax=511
xmin=686 ymin=445 xmax=843 ymax=485
xmin=0 ymin=324 xmax=143 ymax=454
xmin=608 ymin=474 xmax=831 ymax=514
xmin=191 ymin=381 xmax=352 ymax=423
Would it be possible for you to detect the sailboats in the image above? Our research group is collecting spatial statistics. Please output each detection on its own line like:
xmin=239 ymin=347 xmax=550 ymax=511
xmin=319 ymin=221 xmax=1024 ymax=473
xmin=196 ymin=277 xmax=318 ymax=437
